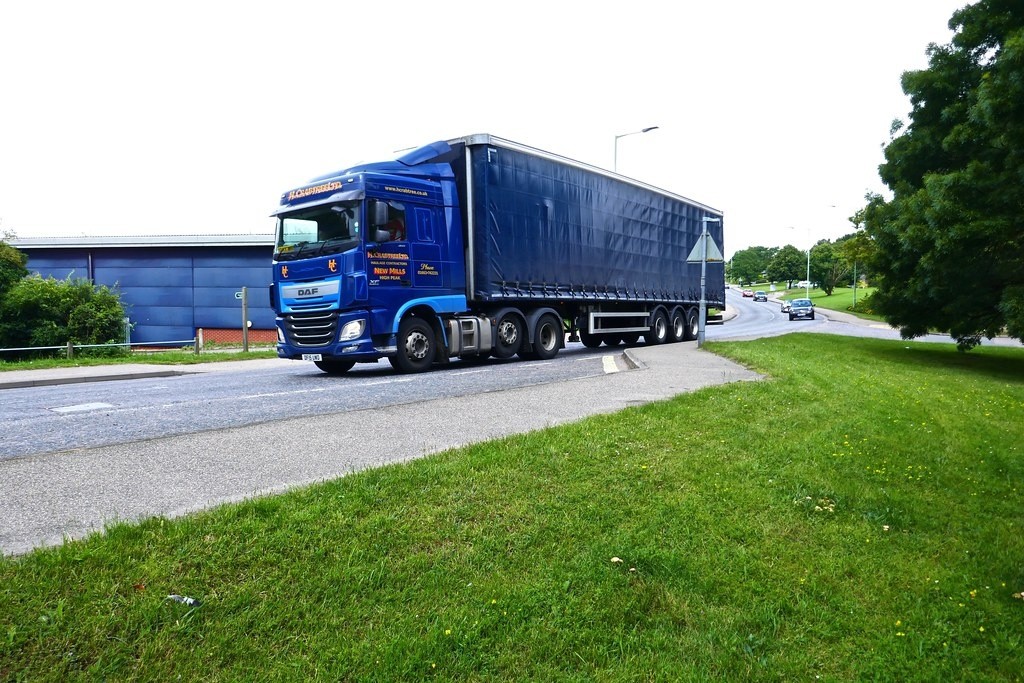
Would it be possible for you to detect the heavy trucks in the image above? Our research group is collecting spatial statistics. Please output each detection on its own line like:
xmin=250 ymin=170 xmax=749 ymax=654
xmin=269 ymin=133 xmax=724 ymax=374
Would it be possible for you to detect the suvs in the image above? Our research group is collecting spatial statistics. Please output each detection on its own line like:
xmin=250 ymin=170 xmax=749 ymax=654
xmin=798 ymin=281 xmax=818 ymax=289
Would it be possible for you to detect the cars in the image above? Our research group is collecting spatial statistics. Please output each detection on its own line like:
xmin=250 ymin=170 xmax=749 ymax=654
xmin=753 ymin=291 xmax=768 ymax=302
xmin=781 ymin=299 xmax=792 ymax=313
xmin=724 ymin=283 xmax=729 ymax=289
xmin=787 ymin=299 xmax=816 ymax=322
xmin=742 ymin=289 xmax=754 ymax=298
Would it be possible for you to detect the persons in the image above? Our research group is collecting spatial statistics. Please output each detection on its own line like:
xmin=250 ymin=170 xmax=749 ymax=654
xmin=377 ymin=211 xmax=404 ymax=242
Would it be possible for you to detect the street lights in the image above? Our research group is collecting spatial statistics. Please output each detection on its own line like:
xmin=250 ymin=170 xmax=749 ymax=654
xmin=831 ymin=205 xmax=856 ymax=308
xmin=614 ymin=126 xmax=659 ymax=171
